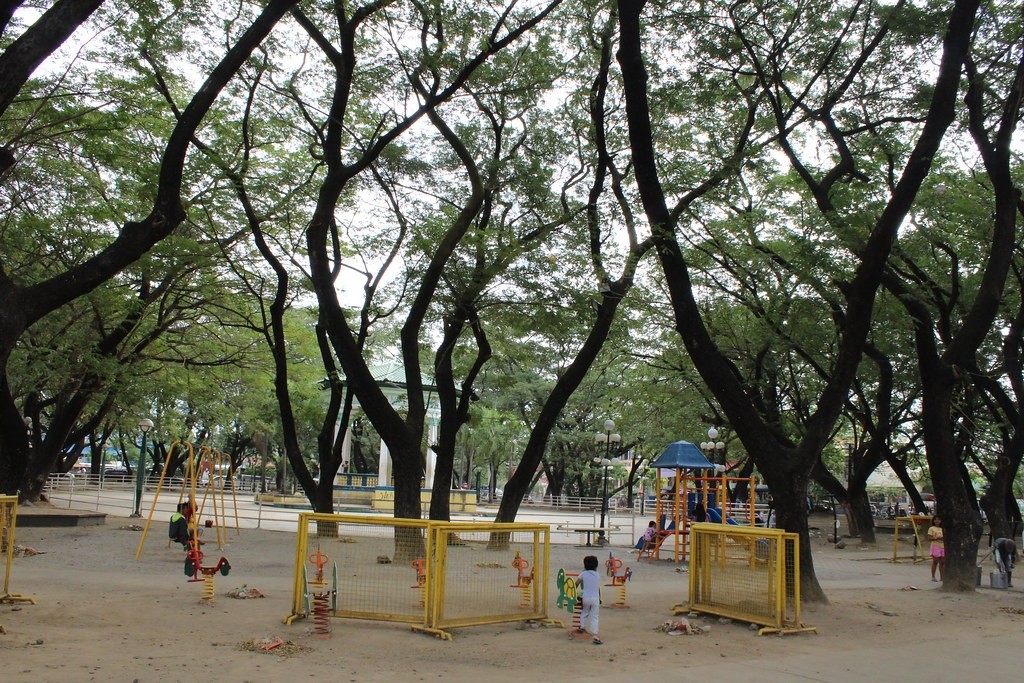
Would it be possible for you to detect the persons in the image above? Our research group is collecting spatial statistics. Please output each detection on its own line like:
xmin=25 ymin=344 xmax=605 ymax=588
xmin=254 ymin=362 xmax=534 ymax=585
xmin=691 ymin=502 xmax=711 ymax=568
xmin=642 ymin=520 xmax=657 ymax=549
xmin=168 ymin=503 xmax=196 ymax=552
xmin=806 ymin=496 xmax=812 ymax=517
xmin=869 ymin=503 xmax=877 ymax=516
xmin=77 ymin=468 xmax=82 ymax=479
xmin=201 ymin=468 xmax=210 ymax=488
xmin=573 ymin=556 xmax=603 ymax=645
xmin=183 ymin=501 xmax=206 ymax=545
xmin=991 ymin=537 xmax=1018 ymax=588
xmin=886 ymin=503 xmax=894 ymax=520
xmin=81 ymin=466 xmax=86 ymax=478
xmin=909 ymin=502 xmax=913 ymax=515
xmin=927 ymin=514 xmax=945 ymax=582
xmin=893 ymin=502 xmax=900 ymax=519
xmin=925 ymin=506 xmax=931 ymax=516
xmin=757 ymin=491 xmax=777 ymax=571
xmin=342 ymin=461 xmax=349 ymax=474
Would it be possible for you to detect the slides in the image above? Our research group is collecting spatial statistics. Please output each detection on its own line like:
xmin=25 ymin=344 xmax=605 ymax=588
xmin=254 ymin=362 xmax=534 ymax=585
xmin=707 ymin=507 xmax=779 ymax=560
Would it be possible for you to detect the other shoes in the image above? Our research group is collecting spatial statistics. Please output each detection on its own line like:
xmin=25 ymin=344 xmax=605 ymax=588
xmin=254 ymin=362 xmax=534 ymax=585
xmin=593 ymin=636 xmax=601 ymax=644
xmin=578 ymin=628 xmax=584 ymax=632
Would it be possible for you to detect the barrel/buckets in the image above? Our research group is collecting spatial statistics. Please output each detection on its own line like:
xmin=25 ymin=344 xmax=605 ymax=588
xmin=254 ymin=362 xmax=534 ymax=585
xmin=989 ymin=572 xmax=1009 ymax=588
xmin=976 ymin=567 xmax=981 ymax=585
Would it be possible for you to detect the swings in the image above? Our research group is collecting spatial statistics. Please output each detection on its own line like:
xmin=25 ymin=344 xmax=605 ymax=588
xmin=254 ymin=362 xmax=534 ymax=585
xmin=171 ymin=445 xmax=192 ymax=546
xmin=205 ymin=453 xmax=219 ymax=529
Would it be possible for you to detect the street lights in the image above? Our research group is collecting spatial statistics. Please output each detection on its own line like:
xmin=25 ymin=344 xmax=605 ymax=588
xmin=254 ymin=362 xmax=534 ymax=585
xmin=598 ymin=420 xmax=619 ymax=544
xmin=700 ymin=426 xmax=725 ymax=490
xmin=133 ymin=417 xmax=156 ymax=519
